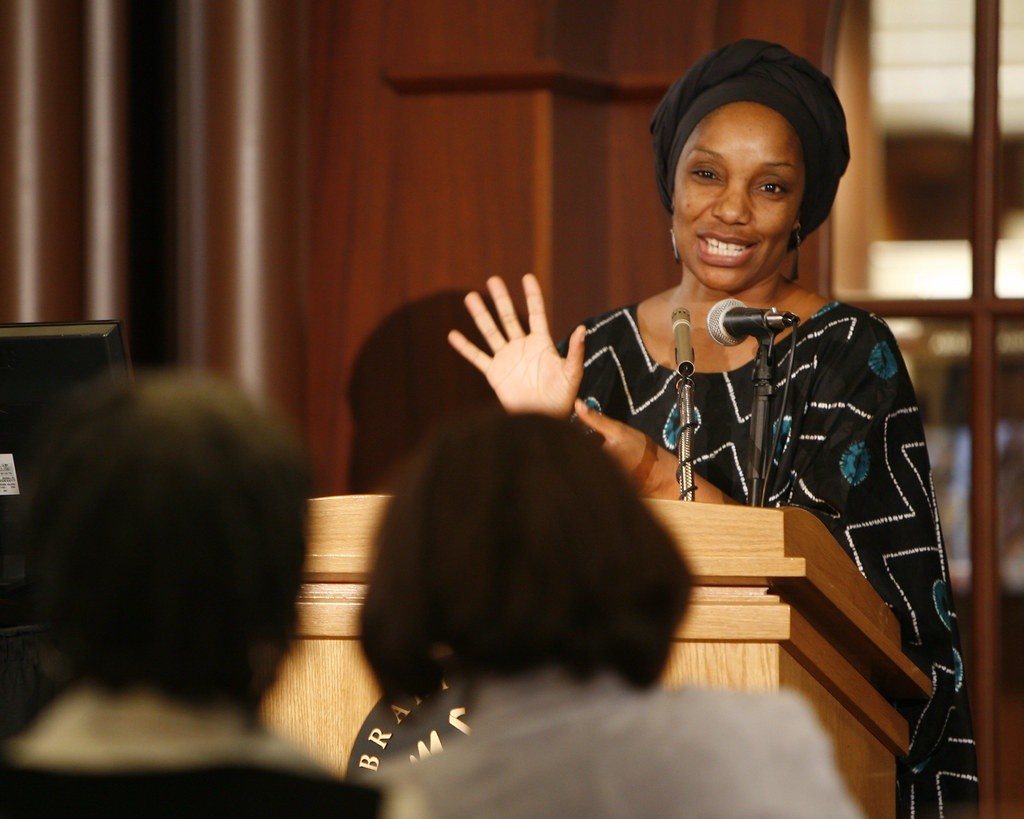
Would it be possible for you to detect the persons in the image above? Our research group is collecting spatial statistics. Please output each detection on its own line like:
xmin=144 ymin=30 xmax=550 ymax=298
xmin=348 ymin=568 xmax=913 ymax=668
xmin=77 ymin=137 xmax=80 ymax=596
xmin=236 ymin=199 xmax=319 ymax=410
xmin=2 ymin=359 xmax=440 ymax=819
xmin=354 ymin=410 xmax=875 ymax=819
xmin=448 ymin=34 xmax=987 ymax=817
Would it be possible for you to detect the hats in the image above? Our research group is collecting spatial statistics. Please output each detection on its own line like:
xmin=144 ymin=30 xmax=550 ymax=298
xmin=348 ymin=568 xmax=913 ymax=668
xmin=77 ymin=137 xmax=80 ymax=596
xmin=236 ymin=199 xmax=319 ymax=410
xmin=649 ymin=39 xmax=850 ymax=249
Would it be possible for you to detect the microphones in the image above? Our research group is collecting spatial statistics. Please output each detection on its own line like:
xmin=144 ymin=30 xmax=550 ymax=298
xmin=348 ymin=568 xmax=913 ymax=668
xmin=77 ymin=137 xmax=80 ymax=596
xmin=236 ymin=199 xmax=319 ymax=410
xmin=706 ymin=298 xmax=799 ymax=347
xmin=672 ymin=308 xmax=695 ymax=378
